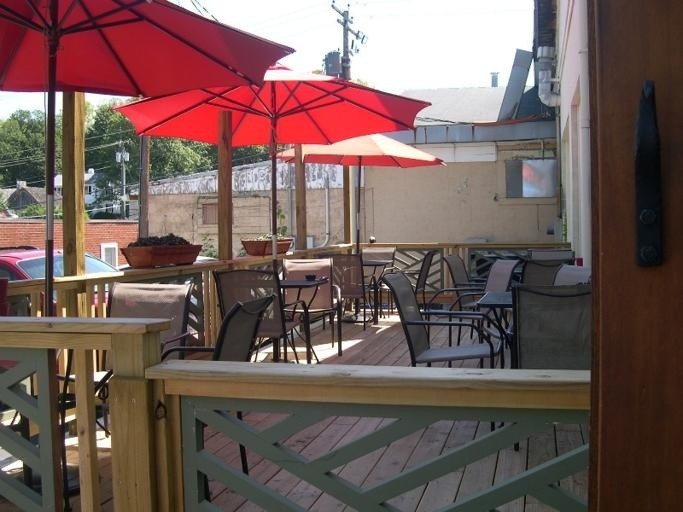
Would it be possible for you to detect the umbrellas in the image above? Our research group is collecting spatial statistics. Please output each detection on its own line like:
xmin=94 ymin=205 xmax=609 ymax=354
xmin=0 ymin=1 xmax=296 ymax=498
xmin=108 ymin=56 xmax=433 ymax=362
xmin=268 ymin=132 xmax=448 ymax=325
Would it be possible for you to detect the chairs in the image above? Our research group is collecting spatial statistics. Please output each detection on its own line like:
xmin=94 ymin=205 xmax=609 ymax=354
xmin=283 ymin=257 xmax=344 ymax=357
xmin=511 ymin=282 xmax=591 ymax=452
xmin=319 ymin=247 xmax=396 ymax=331
xmin=211 ymin=267 xmax=312 ymax=366
xmin=161 ymin=294 xmax=278 ymax=475
xmin=443 ymin=246 xmax=591 ymax=349
xmin=382 ymin=270 xmax=503 ymax=434
xmin=386 ymin=250 xmax=438 ymax=322
xmin=58 ymin=282 xmax=195 ymax=388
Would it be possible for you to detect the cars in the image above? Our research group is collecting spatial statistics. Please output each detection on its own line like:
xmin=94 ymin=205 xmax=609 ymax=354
xmin=114 ymin=255 xmax=219 ymax=309
xmin=0 ymin=245 xmax=198 ymax=372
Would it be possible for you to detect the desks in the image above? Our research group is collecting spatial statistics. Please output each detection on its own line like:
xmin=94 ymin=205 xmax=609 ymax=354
xmin=477 ymin=289 xmax=514 ymax=371
xmin=238 ymin=278 xmax=329 ymax=364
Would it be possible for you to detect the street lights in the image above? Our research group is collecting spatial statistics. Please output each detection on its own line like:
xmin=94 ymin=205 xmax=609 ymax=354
xmin=325 ymin=0 xmax=368 ymax=84
